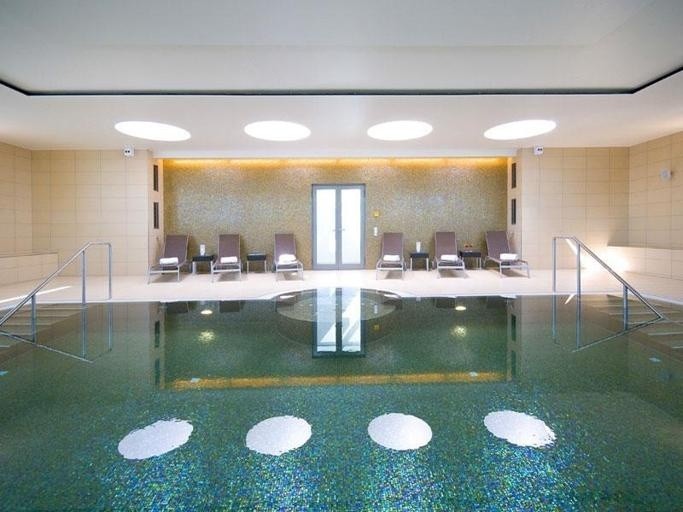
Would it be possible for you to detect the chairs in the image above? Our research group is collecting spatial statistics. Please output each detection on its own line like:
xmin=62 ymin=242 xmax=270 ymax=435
xmin=148 ymin=233 xmax=302 ymax=283
xmin=372 ymin=230 xmax=530 ymax=282
